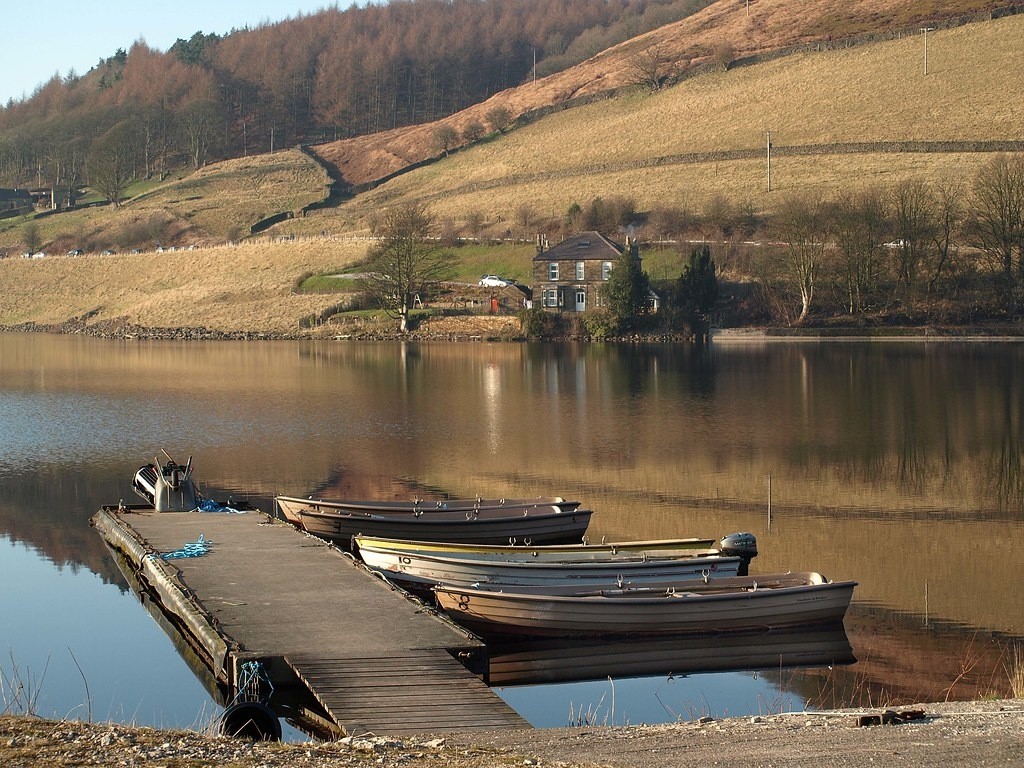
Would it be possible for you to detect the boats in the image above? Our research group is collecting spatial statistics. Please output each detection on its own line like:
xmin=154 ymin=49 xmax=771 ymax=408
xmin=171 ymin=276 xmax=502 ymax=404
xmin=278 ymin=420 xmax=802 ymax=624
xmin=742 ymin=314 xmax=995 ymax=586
xmin=430 ymin=571 xmax=859 ymax=638
xmin=454 ymin=622 xmax=858 ymax=688
xmin=353 ymin=533 xmax=758 ymax=605
xmin=296 ymin=509 xmax=594 ymax=560
xmin=276 ymin=495 xmax=581 ymax=531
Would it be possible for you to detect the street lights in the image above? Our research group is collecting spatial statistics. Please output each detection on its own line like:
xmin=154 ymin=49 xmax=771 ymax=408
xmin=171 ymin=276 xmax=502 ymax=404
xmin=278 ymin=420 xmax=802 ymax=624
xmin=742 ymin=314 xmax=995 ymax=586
xmin=921 ymin=28 xmax=934 ymax=75
xmin=766 ymin=131 xmax=773 ymax=193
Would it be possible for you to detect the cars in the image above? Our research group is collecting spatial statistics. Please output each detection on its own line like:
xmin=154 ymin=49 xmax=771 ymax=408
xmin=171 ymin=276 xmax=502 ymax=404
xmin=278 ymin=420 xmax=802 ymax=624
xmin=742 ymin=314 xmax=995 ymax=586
xmin=99 ymin=250 xmax=116 ymax=255
xmin=23 ymin=252 xmax=33 ymax=258
xmin=884 ymin=239 xmax=912 ymax=248
xmin=32 ymin=252 xmax=46 ymax=258
xmin=156 ymin=247 xmax=167 ymax=253
xmin=66 ymin=250 xmax=84 ymax=257
xmin=478 ymin=276 xmax=513 ymax=288
xmin=129 ymin=248 xmax=143 ymax=254
